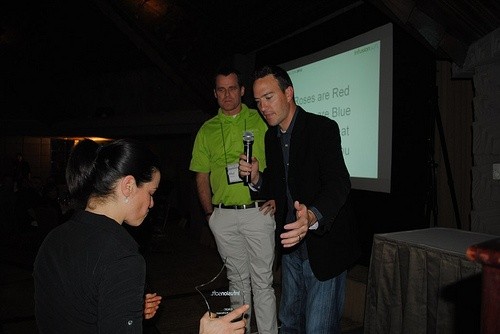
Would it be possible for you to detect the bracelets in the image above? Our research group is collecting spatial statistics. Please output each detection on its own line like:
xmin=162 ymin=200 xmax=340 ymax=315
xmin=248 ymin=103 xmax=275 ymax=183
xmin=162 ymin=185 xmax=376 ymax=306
xmin=205 ymin=212 xmax=213 ymax=216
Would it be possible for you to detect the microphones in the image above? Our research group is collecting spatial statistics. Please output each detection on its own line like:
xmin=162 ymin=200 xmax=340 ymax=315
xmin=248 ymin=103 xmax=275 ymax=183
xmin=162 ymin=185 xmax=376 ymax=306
xmin=243 ymin=131 xmax=255 ymax=186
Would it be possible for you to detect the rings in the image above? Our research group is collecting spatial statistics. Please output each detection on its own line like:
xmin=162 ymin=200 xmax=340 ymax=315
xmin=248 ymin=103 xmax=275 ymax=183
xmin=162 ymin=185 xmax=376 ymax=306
xmin=298 ymin=235 xmax=302 ymax=241
xmin=270 ymin=205 xmax=274 ymax=209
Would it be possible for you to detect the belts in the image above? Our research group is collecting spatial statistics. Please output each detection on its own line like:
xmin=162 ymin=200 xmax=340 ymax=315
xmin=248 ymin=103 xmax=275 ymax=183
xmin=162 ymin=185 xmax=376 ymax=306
xmin=212 ymin=201 xmax=267 ymax=209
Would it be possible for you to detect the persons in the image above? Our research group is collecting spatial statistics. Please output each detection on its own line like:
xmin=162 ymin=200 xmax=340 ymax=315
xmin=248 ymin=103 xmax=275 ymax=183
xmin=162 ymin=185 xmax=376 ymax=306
xmin=189 ymin=66 xmax=278 ymax=334
xmin=238 ymin=66 xmax=354 ymax=334
xmin=34 ymin=138 xmax=250 ymax=334
